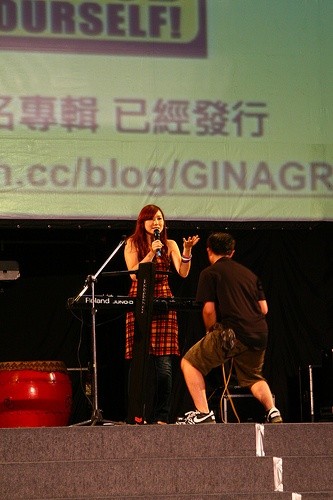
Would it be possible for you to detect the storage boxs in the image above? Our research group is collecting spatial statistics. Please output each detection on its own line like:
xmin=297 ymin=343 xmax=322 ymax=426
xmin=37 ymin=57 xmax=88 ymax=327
xmin=223 ymin=388 xmax=276 ymax=423
xmin=298 ymin=365 xmax=328 ymax=421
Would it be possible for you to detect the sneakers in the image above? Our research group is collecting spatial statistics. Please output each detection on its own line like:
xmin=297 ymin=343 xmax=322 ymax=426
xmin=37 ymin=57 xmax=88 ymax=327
xmin=177 ymin=409 xmax=216 ymax=424
xmin=264 ymin=407 xmax=283 ymax=424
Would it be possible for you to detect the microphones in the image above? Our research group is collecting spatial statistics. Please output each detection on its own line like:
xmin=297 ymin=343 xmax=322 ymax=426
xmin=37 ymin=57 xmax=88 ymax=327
xmin=154 ymin=229 xmax=161 ymax=257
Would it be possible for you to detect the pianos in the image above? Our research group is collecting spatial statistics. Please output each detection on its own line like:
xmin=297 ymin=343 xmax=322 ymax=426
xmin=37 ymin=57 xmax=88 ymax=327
xmin=69 ymin=295 xmax=205 ymax=311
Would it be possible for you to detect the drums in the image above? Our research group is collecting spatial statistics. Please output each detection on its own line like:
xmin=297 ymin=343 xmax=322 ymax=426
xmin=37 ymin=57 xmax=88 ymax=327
xmin=0 ymin=360 xmax=73 ymax=428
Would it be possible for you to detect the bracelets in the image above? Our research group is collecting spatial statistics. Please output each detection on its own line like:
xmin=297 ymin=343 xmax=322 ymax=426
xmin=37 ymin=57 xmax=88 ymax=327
xmin=182 ymin=254 xmax=192 ymax=263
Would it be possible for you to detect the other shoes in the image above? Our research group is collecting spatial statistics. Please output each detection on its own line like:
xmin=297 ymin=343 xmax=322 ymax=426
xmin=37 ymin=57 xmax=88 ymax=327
xmin=157 ymin=417 xmax=169 ymax=424
xmin=137 ymin=418 xmax=147 ymax=425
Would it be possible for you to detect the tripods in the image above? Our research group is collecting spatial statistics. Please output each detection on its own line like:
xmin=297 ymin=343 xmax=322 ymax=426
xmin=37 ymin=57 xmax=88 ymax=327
xmin=69 ymin=235 xmax=127 ymax=426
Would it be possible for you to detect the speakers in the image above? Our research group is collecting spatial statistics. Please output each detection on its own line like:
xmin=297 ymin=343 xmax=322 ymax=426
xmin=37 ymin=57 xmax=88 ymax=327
xmin=299 ymin=364 xmax=333 ymax=423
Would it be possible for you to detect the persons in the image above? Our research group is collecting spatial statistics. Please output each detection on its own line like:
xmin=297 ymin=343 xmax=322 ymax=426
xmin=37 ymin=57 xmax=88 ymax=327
xmin=175 ymin=232 xmax=283 ymax=424
xmin=123 ymin=204 xmax=200 ymax=425
